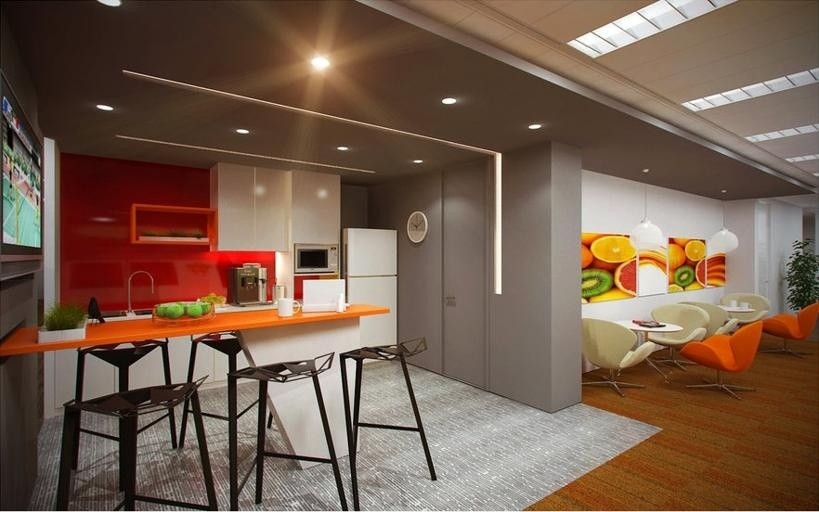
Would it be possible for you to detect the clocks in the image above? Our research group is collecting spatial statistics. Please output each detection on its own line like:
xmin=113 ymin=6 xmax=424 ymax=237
xmin=405 ymin=210 xmax=429 ymax=244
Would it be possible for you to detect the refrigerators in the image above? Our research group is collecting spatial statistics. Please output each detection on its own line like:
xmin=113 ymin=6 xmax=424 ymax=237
xmin=341 ymin=227 xmax=398 ymax=350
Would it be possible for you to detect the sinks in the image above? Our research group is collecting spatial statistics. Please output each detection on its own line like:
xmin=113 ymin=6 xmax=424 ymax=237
xmin=134 ymin=309 xmax=153 ymax=318
xmin=87 ymin=311 xmax=128 ymax=321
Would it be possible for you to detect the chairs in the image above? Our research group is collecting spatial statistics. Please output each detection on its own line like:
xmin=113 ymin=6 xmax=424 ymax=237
xmin=679 ymin=319 xmax=765 ymax=401
xmin=760 ymin=301 xmax=819 ymax=360
xmin=648 ymin=303 xmax=709 ymax=371
xmin=720 ymin=292 xmax=770 ymax=327
xmin=677 ymin=301 xmax=739 ymax=337
xmin=581 ymin=317 xmax=656 ymax=395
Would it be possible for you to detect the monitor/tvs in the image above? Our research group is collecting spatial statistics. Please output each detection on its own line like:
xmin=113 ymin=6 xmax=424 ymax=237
xmin=0 ymin=73 xmax=43 ymax=266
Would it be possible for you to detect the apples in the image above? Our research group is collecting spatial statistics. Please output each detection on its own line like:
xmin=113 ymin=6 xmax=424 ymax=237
xmin=157 ymin=302 xmax=211 ymax=319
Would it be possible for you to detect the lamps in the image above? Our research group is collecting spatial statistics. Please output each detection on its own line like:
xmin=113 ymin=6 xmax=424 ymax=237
xmin=707 ymin=187 xmax=743 ymax=256
xmin=628 ymin=165 xmax=666 ymax=252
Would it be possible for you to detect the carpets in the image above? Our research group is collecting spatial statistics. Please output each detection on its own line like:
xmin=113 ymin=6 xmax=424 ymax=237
xmin=41 ymin=350 xmax=666 ymax=512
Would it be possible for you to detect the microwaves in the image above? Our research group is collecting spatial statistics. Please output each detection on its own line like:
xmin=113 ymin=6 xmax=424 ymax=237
xmin=294 ymin=242 xmax=339 ymax=274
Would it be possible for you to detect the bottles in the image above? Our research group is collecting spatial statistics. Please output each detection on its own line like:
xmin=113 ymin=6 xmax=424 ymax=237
xmin=336 ymin=292 xmax=343 ymax=313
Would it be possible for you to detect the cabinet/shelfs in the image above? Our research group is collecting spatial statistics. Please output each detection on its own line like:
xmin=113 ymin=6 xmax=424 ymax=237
xmin=209 ymin=161 xmax=293 ymax=254
xmin=51 ymin=338 xmax=168 ymax=410
xmin=166 ymin=334 xmax=259 ymax=386
xmin=128 ymin=204 xmax=216 ymax=247
xmin=289 ymin=171 xmax=341 ymax=245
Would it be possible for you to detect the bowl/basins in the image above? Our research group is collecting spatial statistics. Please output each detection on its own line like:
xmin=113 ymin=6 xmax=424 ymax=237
xmin=151 ymin=301 xmax=216 ymax=325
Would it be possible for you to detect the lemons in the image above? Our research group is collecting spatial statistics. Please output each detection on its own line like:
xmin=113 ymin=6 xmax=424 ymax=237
xmin=685 ymin=281 xmax=704 ymax=291
xmin=589 ymin=287 xmax=634 ymax=303
xmin=200 ymin=292 xmax=225 ymax=304
xmin=669 ymin=284 xmax=684 ymax=294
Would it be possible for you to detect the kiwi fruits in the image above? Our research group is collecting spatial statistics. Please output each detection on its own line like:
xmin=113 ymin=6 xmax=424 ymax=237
xmin=582 ymin=269 xmax=613 ymax=298
xmin=674 ymin=265 xmax=695 ymax=286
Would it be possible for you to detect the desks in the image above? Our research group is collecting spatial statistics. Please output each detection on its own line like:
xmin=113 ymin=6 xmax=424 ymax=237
xmin=613 ymin=320 xmax=684 ymax=384
xmin=714 ymin=302 xmax=756 ymax=317
xmin=2 ymin=301 xmax=394 ymax=471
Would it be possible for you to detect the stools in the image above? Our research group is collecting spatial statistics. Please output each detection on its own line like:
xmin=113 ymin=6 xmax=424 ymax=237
xmin=223 ymin=349 xmax=349 ymax=510
xmin=338 ymin=332 xmax=440 ymax=512
xmin=71 ymin=333 xmax=179 ymax=456
xmin=178 ymin=329 xmax=275 ymax=449
xmin=52 ymin=372 xmax=220 ymax=511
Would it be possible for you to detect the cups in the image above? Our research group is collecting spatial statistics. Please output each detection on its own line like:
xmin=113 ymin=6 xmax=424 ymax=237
xmin=277 ymin=298 xmax=301 ymax=317
xmin=740 ymin=302 xmax=753 ymax=311
xmin=272 ymin=285 xmax=286 ymax=307
xmin=728 ymin=300 xmax=737 ymax=309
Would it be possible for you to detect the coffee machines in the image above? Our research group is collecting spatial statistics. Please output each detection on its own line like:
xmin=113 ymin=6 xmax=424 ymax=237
xmin=232 ymin=263 xmax=269 ymax=307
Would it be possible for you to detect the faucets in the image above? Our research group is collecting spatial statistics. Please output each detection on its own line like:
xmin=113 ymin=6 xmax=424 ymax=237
xmin=128 ymin=271 xmax=154 ymax=311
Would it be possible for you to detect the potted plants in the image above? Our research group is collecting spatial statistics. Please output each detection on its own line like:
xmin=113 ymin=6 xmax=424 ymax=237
xmin=34 ymin=303 xmax=91 ymax=344
